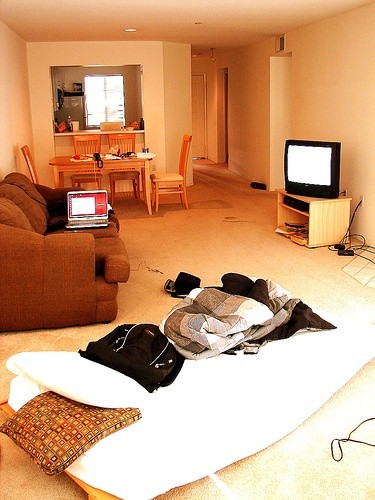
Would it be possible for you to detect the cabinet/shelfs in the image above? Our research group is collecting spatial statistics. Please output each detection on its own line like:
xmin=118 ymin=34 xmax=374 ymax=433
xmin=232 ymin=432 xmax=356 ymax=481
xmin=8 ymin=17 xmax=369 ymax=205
xmin=274 ymin=188 xmax=351 ymax=248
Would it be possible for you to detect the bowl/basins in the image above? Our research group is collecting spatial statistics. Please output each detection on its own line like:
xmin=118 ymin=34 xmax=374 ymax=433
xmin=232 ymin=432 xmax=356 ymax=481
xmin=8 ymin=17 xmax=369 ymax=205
xmin=126 ymin=127 xmax=134 ymax=130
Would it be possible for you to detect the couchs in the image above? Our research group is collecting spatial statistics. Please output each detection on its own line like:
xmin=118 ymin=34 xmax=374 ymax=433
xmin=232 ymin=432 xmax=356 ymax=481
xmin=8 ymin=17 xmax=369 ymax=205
xmin=0 ymin=172 xmax=132 ymax=336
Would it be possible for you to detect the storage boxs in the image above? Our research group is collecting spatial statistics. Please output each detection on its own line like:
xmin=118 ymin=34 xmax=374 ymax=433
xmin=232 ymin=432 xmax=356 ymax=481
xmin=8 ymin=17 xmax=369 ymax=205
xmin=100 ymin=121 xmax=124 ymax=131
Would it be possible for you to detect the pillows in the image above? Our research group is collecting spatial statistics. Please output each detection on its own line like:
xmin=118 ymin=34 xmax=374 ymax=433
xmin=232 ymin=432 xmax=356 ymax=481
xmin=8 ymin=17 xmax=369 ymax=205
xmin=11 ymin=350 xmax=156 ymax=408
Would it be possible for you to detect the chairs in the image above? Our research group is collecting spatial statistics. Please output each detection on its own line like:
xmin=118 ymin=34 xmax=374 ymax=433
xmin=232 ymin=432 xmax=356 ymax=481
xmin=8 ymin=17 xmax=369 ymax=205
xmin=21 ymin=130 xmax=194 ymax=215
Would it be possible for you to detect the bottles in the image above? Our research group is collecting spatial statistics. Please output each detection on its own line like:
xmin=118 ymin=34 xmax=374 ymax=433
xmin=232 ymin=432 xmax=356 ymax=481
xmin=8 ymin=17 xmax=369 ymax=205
xmin=140 ymin=118 xmax=144 ymax=130
xmin=68 ymin=122 xmax=73 ymax=132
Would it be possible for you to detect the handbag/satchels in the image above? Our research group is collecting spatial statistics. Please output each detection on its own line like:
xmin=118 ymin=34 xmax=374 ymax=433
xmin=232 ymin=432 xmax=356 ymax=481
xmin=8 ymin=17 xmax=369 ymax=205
xmin=78 ymin=323 xmax=185 ymax=393
xmin=164 ymin=272 xmax=201 ymax=295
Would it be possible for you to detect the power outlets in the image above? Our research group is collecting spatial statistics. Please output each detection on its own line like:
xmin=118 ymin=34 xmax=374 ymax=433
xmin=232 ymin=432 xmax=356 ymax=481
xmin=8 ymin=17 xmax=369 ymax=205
xmin=359 ymin=195 xmax=365 ymax=208
xmin=340 ymin=189 xmax=347 ymax=197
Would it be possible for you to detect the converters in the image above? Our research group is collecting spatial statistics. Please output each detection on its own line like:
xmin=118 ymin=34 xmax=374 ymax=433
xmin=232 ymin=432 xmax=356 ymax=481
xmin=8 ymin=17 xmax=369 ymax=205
xmin=334 ymin=244 xmax=345 ymax=250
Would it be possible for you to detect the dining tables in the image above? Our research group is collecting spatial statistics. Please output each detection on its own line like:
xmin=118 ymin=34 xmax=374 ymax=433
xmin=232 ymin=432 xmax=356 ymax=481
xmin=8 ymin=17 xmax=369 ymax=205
xmin=47 ymin=152 xmax=153 ymax=215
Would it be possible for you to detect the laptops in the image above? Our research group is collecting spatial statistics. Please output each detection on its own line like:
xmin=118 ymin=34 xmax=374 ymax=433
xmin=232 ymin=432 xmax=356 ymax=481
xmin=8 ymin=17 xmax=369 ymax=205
xmin=65 ymin=190 xmax=109 ymax=228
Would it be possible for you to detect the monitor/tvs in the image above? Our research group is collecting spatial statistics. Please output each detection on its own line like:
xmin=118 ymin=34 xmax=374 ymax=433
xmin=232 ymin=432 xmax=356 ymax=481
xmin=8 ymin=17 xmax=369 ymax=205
xmin=284 ymin=140 xmax=342 ymax=199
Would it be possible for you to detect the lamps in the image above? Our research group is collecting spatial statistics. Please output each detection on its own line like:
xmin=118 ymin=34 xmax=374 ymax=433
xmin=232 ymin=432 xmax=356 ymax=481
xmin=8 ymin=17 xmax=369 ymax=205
xmin=208 ymin=47 xmax=217 ymax=62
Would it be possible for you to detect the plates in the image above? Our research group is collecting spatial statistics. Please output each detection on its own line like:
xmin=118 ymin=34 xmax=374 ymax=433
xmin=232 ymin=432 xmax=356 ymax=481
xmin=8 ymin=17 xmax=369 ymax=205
xmin=71 ymin=153 xmax=155 ymax=162
